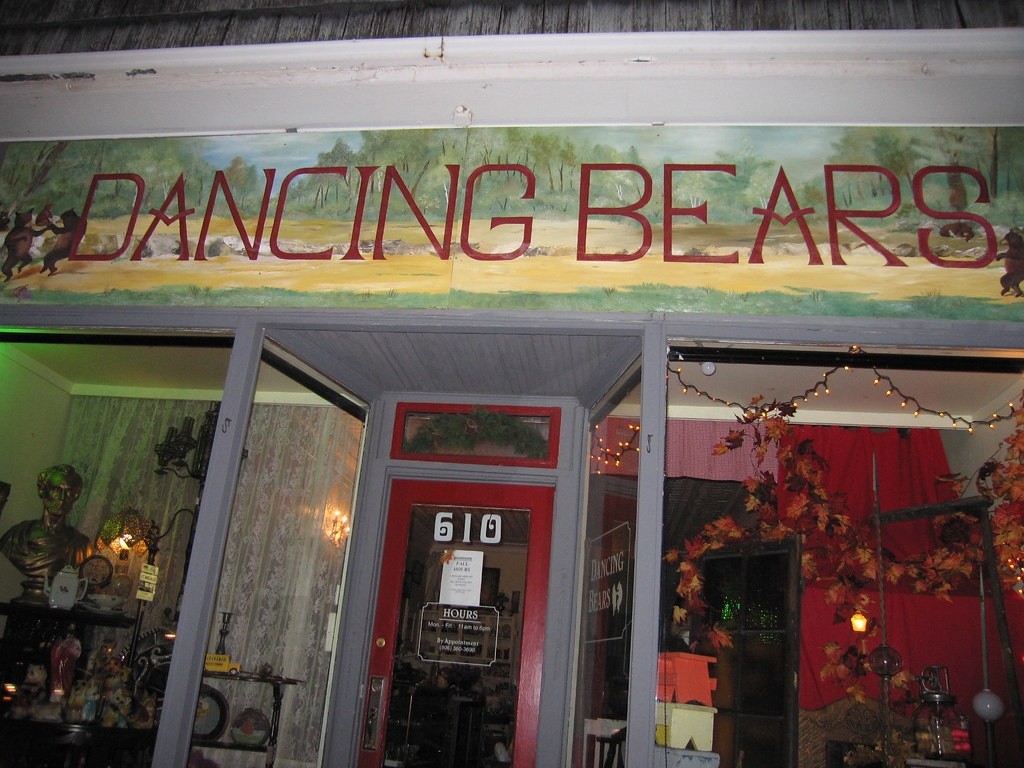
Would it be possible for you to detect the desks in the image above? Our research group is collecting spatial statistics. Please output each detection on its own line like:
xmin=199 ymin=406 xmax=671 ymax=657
xmin=0 ymin=601 xmax=136 ymax=660
xmin=149 ymin=665 xmax=302 ymax=768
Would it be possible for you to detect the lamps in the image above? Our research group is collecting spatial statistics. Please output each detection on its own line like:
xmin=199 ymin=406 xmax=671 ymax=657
xmin=154 ymin=414 xmax=199 ymax=480
xmin=99 ymin=505 xmax=151 ymax=601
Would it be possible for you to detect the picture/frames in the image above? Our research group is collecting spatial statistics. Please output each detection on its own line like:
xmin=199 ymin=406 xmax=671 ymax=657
xmin=390 ymin=401 xmax=562 ymax=469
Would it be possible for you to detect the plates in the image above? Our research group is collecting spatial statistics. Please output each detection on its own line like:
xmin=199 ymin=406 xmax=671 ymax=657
xmin=84 ymin=605 xmax=125 ymax=615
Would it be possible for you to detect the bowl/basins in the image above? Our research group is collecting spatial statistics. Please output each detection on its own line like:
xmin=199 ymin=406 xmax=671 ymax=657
xmin=88 ymin=594 xmax=129 ymax=611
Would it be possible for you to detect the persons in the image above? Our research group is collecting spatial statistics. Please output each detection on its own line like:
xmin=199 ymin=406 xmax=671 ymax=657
xmin=0 ymin=463 xmax=93 ymax=585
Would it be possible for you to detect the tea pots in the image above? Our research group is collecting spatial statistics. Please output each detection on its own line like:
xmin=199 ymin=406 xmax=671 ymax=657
xmin=43 ymin=566 xmax=88 ymax=610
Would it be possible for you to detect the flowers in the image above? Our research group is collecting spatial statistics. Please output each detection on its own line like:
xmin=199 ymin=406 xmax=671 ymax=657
xmin=666 ymin=406 xmax=1024 ymax=649
